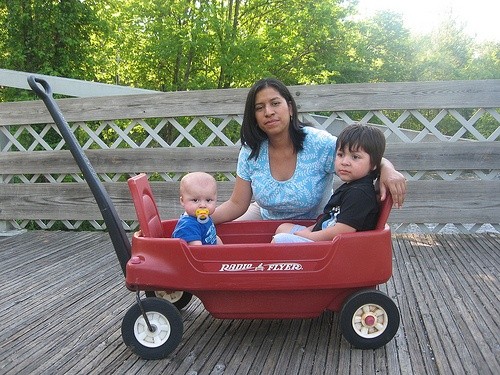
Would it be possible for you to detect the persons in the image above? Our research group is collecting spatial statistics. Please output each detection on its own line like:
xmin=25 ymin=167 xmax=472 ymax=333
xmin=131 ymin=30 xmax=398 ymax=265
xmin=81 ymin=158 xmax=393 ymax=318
xmin=209 ymin=77 xmax=406 ymax=224
xmin=171 ymin=170 xmax=225 ymax=245
xmin=269 ymin=123 xmax=386 ymax=244
